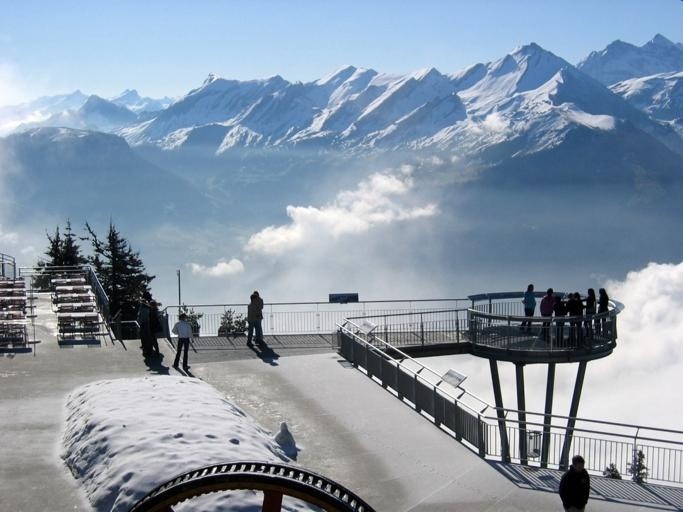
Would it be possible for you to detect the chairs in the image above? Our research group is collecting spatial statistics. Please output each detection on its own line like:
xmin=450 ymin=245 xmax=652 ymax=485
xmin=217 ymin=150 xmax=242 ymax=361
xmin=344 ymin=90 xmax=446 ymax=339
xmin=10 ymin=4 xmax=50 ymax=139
xmin=0 ymin=277 xmax=102 ymax=350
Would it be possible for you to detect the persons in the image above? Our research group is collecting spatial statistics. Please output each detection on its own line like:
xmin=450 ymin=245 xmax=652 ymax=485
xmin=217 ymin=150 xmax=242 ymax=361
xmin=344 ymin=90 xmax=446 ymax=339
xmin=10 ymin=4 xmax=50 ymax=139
xmin=246 ymin=294 xmax=258 ymax=347
xmin=137 ymin=292 xmax=193 ymax=369
xmin=520 ymin=283 xmax=536 ymax=331
xmin=539 ymin=288 xmax=609 ymax=346
xmin=253 ymin=290 xmax=264 ymax=344
xmin=558 ymin=455 xmax=592 ymax=512
